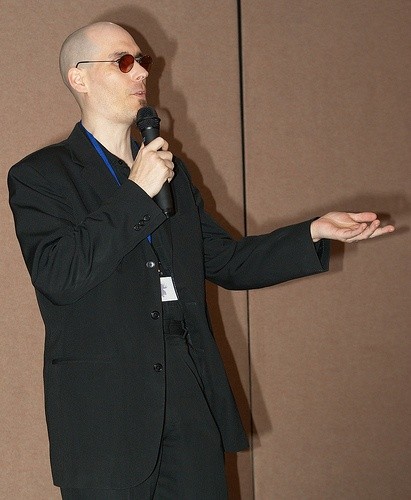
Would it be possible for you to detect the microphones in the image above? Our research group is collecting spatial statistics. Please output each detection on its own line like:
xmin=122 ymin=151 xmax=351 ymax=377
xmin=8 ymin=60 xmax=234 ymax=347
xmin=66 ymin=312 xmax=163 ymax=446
xmin=136 ymin=107 xmax=176 ymax=217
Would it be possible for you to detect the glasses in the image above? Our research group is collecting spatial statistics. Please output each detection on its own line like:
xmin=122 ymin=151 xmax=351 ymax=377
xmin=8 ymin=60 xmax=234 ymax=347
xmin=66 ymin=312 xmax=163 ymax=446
xmin=76 ymin=54 xmax=154 ymax=73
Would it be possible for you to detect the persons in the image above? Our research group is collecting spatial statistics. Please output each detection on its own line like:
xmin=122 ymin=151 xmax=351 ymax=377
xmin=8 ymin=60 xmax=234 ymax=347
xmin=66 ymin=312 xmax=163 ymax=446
xmin=7 ymin=22 xmax=396 ymax=500
